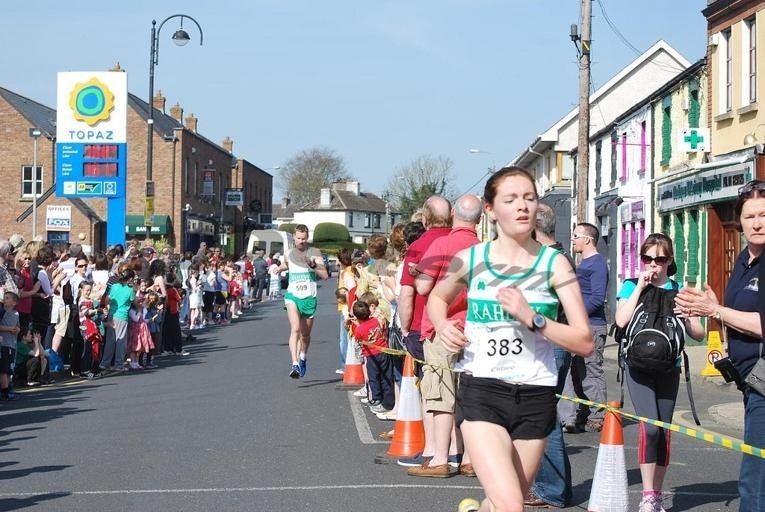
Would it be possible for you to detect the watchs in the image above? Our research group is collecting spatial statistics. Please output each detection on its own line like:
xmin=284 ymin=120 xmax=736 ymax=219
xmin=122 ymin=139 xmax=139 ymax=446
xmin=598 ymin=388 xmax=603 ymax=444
xmin=527 ymin=312 xmax=546 ymax=332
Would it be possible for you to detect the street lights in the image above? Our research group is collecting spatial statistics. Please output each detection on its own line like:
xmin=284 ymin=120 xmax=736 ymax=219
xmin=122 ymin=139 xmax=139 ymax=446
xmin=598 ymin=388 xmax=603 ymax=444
xmin=144 ymin=13 xmax=202 ymax=238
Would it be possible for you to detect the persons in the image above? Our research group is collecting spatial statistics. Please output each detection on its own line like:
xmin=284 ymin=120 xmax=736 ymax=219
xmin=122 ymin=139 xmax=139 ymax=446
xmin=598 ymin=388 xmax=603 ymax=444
xmin=522 ymin=203 xmax=575 ymax=508
xmin=673 ymin=180 xmax=765 ymax=512
xmin=274 ymin=224 xmax=328 ymax=378
xmin=337 ymin=196 xmax=482 ymax=478
xmin=616 ymin=233 xmax=705 ymax=511
xmin=427 ymin=167 xmax=594 ymax=511
xmin=189 ymin=242 xmax=282 ymax=329
xmin=0 ymin=235 xmax=192 ymax=401
xmin=562 ymin=223 xmax=610 ymax=433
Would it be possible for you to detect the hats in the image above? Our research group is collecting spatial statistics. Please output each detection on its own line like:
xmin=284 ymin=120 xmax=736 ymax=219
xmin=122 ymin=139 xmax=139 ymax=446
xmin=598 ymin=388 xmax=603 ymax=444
xmin=142 ymin=247 xmax=156 ymax=254
xmin=9 ymin=234 xmax=24 ymax=255
xmin=69 ymin=245 xmax=82 ymax=257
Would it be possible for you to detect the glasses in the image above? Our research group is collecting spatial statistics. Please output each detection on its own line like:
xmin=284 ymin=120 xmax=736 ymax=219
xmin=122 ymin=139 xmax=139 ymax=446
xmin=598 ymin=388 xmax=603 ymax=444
xmin=641 ymin=253 xmax=670 ymax=267
xmin=573 ymin=233 xmax=595 ymax=240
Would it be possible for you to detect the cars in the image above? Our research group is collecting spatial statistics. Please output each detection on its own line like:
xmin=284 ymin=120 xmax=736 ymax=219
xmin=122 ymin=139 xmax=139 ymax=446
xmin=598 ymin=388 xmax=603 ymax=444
xmin=323 ymin=253 xmax=332 ymax=277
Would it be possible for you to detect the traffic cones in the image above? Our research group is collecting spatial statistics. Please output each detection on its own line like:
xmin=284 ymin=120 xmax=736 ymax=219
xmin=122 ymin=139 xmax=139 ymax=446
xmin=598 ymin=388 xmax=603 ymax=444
xmin=386 ymin=353 xmax=425 ymax=457
xmin=334 ymin=329 xmax=365 ymax=391
xmin=587 ymin=402 xmax=631 ymax=512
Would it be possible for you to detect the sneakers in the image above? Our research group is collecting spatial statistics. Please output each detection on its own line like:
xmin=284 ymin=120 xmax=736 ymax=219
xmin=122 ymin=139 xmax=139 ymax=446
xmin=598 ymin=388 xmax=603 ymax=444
xmin=561 ymin=414 xmax=602 ymax=433
xmin=638 ymin=489 xmax=668 ymax=511
xmin=353 ymin=385 xmax=396 ymax=420
xmin=459 ymin=498 xmax=480 ymax=512
xmin=398 ymin=451 xmax=477 ymax=477
xmin=290 ymin=359 xmax=306 ymax=378
xmin=336 ymin=368 xmax=344 ymax=373
xmin=71 ymin=349 xmax=189 ymax=378
xmin=180 ymin=304 xmax=253 ymax=330
xmin=0 ymin=376 xmax=55 ymax=402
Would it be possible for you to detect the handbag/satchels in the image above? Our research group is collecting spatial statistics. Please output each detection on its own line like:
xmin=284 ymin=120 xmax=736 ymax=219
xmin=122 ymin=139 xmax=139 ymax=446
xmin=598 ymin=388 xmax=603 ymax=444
xmin=389 ymin=328 xmax=407 ymax=359
xmin=745 ymin=358 xmax=765 ymax=398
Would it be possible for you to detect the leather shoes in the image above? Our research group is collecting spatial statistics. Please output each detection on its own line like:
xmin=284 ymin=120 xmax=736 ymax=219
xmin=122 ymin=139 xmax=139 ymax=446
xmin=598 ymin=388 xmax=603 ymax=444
xmin=524 ymin=490 xmax=561 ymax=508
xmin=379 ymin=432 xmax=393 ymax=437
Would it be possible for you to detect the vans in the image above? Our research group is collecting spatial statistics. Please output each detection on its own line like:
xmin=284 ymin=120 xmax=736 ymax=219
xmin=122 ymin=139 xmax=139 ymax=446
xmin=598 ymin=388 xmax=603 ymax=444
xmin=246 ymin=229 xmax=295 ymax=289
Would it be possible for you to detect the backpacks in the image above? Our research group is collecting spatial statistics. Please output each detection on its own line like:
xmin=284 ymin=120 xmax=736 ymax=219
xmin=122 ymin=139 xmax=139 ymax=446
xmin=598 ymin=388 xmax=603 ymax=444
xmin=614 ymin=279 xmax=685 ymax=380
